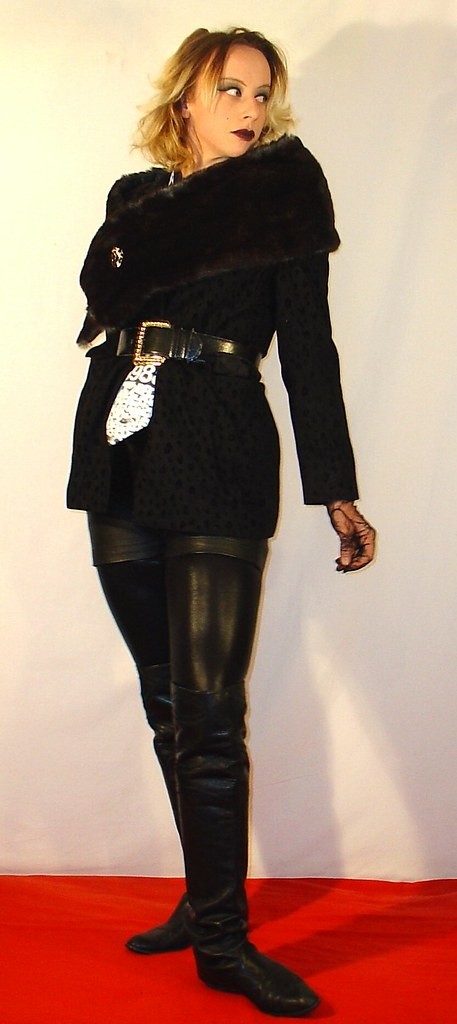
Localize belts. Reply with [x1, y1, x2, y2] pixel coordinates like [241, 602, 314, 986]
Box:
[104, 323, 263, 372]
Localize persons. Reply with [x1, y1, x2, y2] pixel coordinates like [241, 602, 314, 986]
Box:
[67, 29, 375, 1017]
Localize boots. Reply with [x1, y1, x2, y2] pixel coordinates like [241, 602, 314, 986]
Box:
[126, 666, 193, 954]
[172, 682, 320, 1017]
